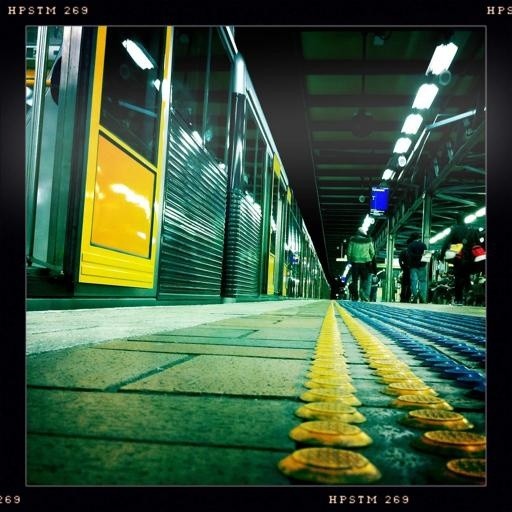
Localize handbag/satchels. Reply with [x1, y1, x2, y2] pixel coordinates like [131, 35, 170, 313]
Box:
[444, 242, 464, 262]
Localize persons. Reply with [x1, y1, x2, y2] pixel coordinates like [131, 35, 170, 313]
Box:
[441, 213, 473, 305]
[398, 239, 413, 302]
[347, 227, 375, 301]
[407, 231, 429, 303]
